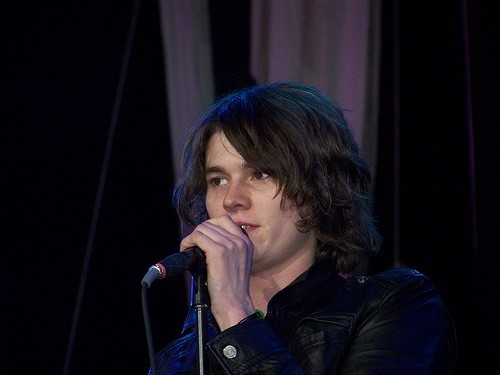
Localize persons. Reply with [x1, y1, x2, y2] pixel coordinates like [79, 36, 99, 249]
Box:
[148, 80, 443, 375]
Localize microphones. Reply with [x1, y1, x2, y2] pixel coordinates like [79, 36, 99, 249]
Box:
[142, 245, 205, 286]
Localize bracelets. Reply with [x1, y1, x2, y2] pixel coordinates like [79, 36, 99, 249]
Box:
[214, 309, 264, 342]
